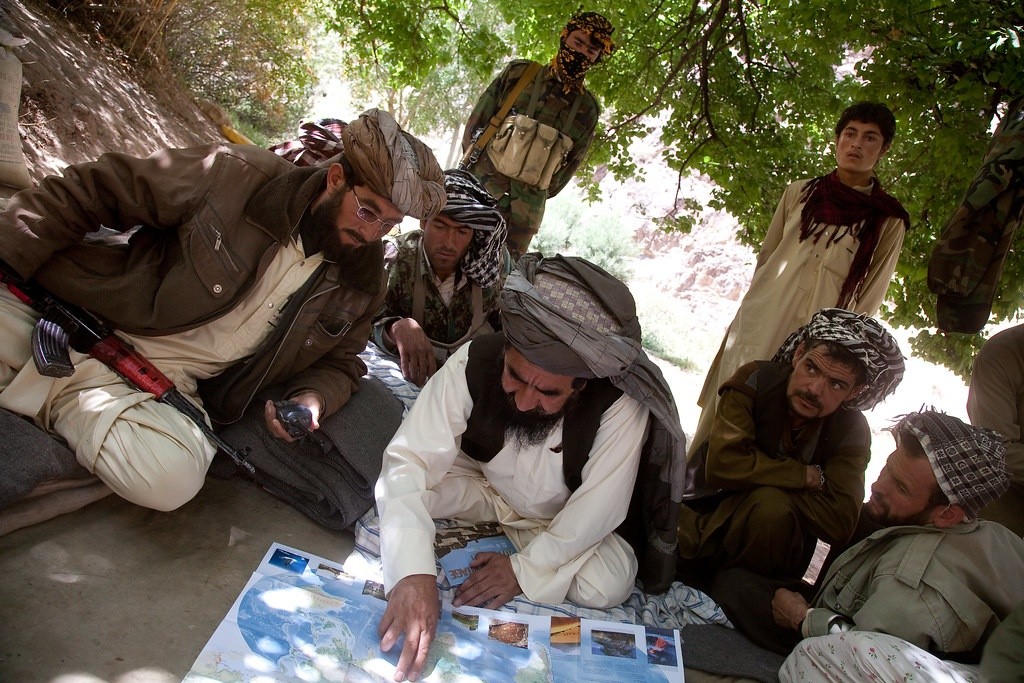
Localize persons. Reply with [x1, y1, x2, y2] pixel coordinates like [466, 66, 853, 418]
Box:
[373, 251, 687, 682]
[456, 12, 621, 263]
[965, 319, 1024, 488]
[687, 102, 908, 501]
[0, 107, 448, 514]
[773, 411, 1023, 682]
[672, 305, 905, 655]
[366, 169, 516, 389]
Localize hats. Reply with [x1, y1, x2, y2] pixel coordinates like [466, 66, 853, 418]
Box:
[341, 107, 448, 220]
[501, 251, 643, 380]
[568, 12, 615, 55]
[891, 411, 1013, 524]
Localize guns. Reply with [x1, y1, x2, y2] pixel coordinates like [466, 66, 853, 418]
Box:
[0, 259, 256, 475]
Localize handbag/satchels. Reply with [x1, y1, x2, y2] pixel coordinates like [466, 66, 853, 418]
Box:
[486, 66, 584, 191]
